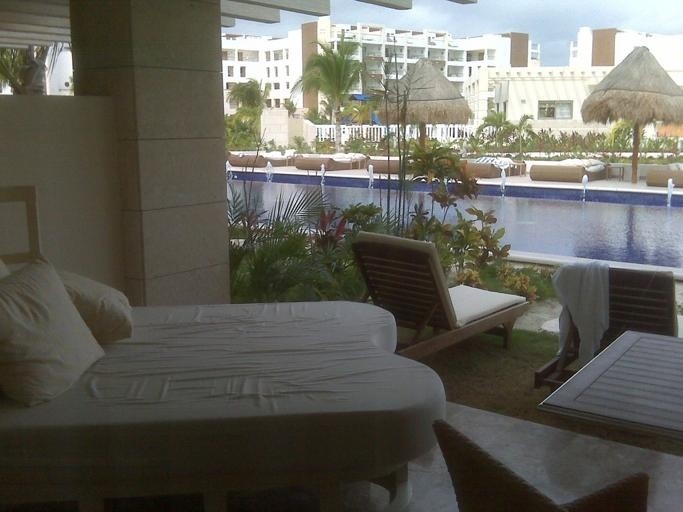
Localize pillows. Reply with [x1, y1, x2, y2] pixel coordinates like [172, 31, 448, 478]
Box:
[284, 149, 295, 157]
[0, 252, 134, 408]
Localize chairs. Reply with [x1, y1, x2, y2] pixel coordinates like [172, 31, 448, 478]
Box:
[533, 262, 679, 392]
[350, 229, 531, 360]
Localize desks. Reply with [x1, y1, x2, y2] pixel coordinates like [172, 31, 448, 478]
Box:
[535, 328, 683, 440]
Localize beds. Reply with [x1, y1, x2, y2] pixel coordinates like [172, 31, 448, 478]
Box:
[0, 299, 447, 512]
[227, 154, 683, 188]
